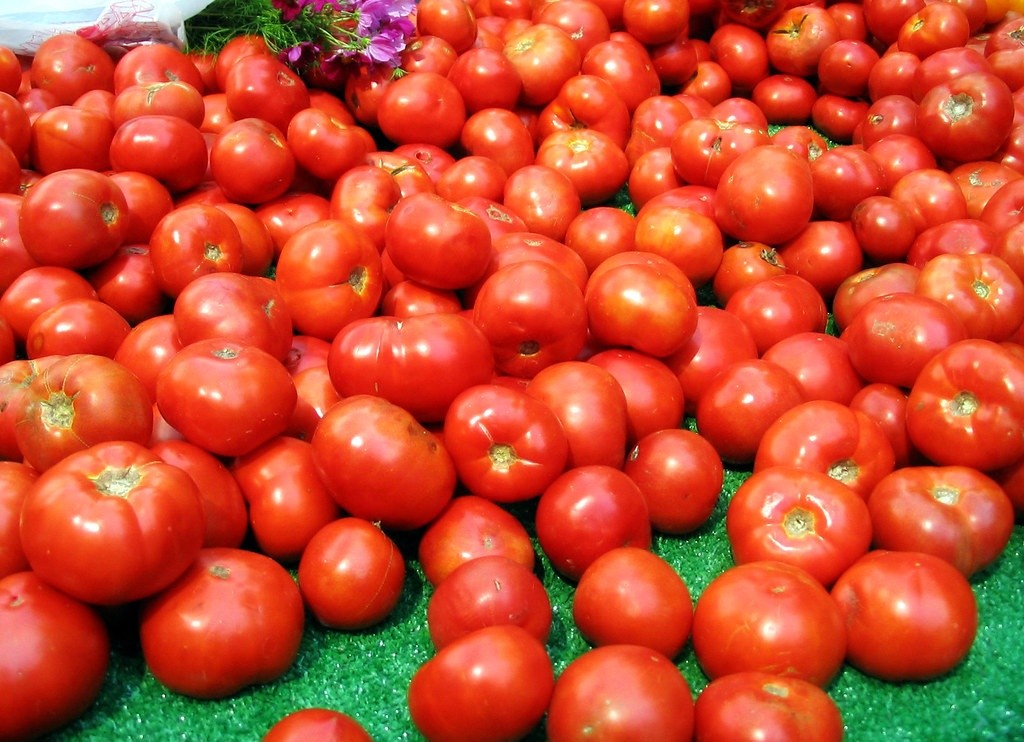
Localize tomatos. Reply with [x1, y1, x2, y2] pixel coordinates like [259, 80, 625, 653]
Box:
[0, 0, 1024, 742]
